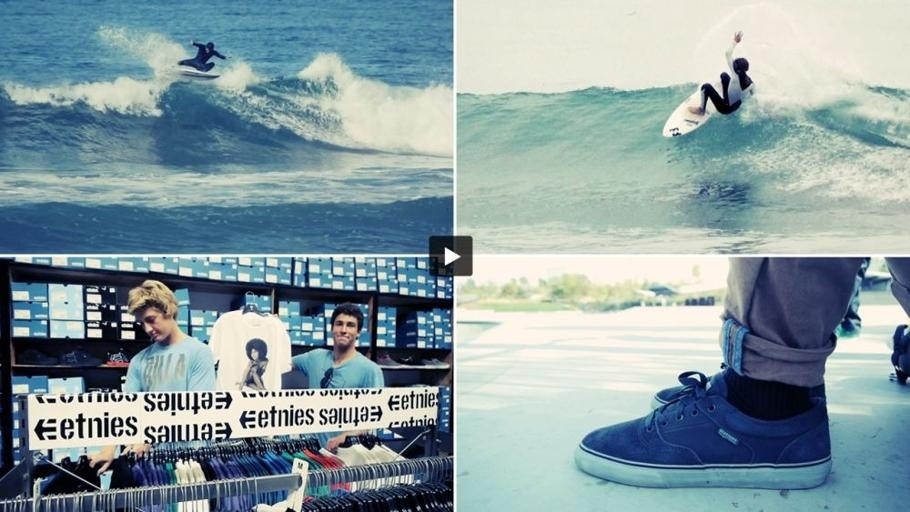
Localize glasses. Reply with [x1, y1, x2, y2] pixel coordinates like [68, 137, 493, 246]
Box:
[320, 368, 333, 388]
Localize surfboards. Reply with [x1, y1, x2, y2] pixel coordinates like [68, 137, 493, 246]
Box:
[662, 79, 725, 138]
[170, 69, 219, 81]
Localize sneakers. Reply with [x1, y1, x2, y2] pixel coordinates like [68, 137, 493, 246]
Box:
[574, 371, 833, 490]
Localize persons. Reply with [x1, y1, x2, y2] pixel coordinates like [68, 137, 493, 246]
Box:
[570, 257, 910, 488]
[287, 299, 388, 453]
[688, 30, 755, 117]
[89, 275, 217, 474]
[236, 338, 269, 390]
[175, 40, 225, 72]
